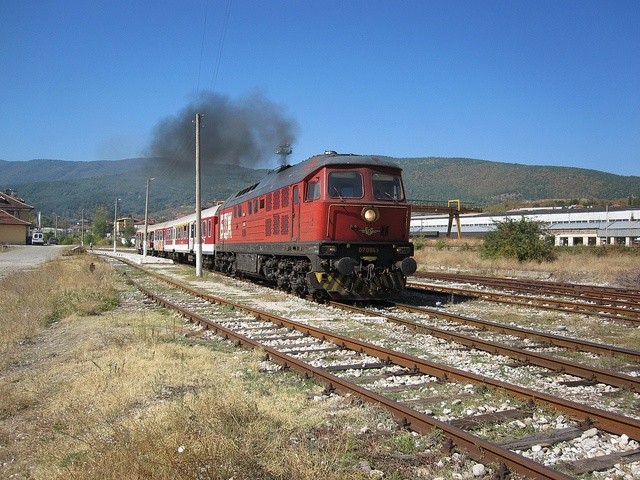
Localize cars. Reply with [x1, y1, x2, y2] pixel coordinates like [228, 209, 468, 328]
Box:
[47, 237, 58, 245]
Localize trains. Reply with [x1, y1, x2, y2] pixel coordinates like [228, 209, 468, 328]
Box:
[135, 151, 418, 305]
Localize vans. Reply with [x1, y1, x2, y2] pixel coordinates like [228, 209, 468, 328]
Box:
[32, 233, 44, 245]
